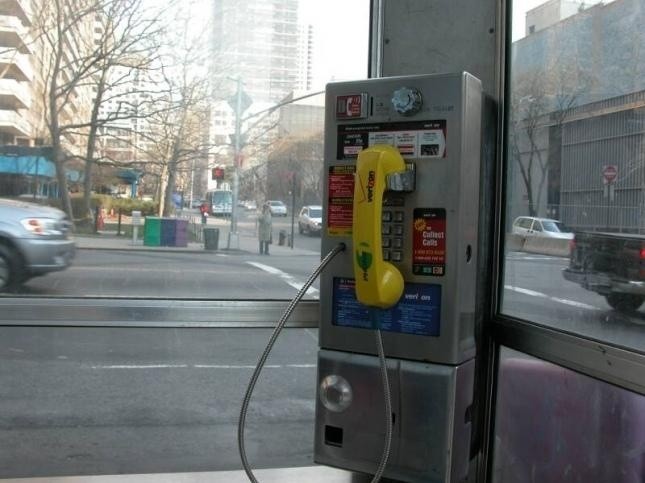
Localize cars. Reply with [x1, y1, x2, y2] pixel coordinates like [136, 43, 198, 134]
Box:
[1, 197, 77, 289]
[239, 198, 257, 210]
[299, 205, 322, 233]
[263, 201, 288, 216]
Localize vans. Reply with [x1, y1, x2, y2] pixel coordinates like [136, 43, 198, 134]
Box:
[514, 216, 576, 240]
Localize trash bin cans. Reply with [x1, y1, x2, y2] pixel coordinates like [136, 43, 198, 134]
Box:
[160, 217, 176, 247]
[203, 228, 219, 250]
[174, 219, 189, 247]
[143, 215, 161, 246]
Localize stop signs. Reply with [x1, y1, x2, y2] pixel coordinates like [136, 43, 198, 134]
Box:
[604, 165, 618, 184]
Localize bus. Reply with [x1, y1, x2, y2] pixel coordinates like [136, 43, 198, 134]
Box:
[206, 189, 233, 215]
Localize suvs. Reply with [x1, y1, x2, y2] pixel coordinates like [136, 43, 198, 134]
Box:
[561, 230, 644, 310]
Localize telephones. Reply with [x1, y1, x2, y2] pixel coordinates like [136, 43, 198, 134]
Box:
[317, 69, 498, 365]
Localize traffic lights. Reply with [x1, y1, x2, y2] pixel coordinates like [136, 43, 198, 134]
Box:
[213, 169, 223, 177]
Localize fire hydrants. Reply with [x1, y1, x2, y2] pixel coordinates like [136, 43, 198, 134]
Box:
[278, 230, 285, 245]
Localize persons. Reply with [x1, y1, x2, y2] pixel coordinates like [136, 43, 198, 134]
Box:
[200, 201, 208, 225]
[257, 199, 273, 255]
